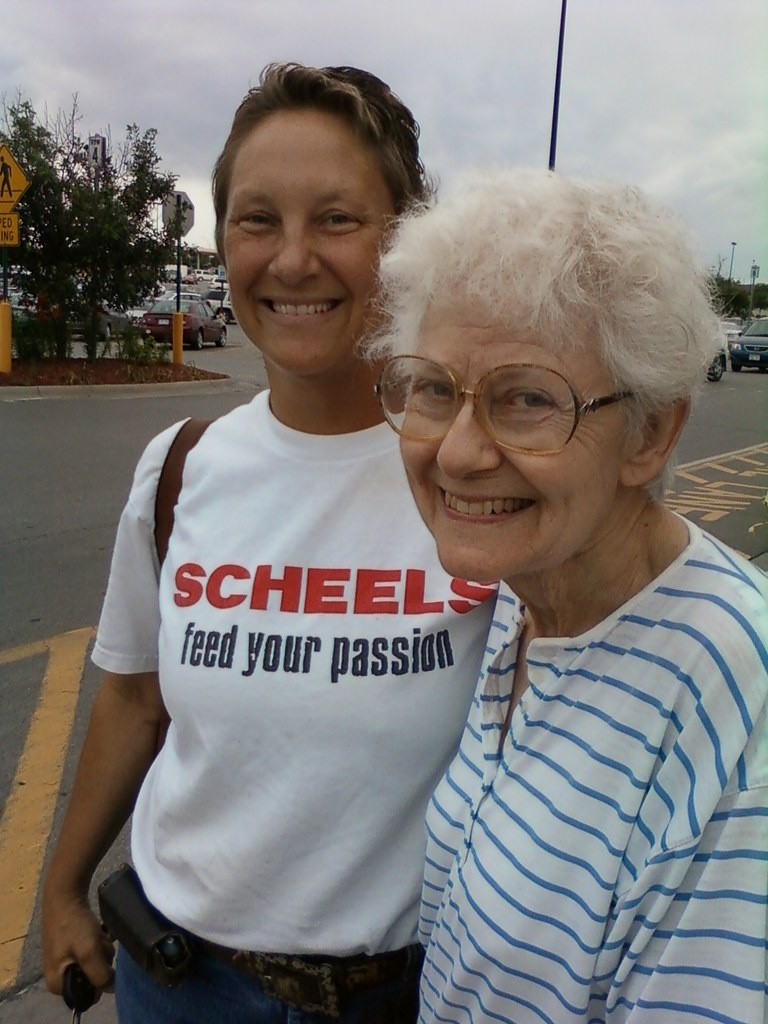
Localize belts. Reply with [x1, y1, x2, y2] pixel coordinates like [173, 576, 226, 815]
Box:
[172, 916, 431, 1019]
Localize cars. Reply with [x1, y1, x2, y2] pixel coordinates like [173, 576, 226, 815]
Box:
[125, 298, 161, 332]
[139, 299, 228, 349]
[182, 275, 197, 284]
[163, 292, 201, 315]
[707, 349, 727, 382]
[0, 265, 132, 342]
[199, 288, 236, 325]
[721, 322, 743, 350]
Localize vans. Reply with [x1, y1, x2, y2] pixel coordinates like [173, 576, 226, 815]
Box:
[191, 270, 218, 282]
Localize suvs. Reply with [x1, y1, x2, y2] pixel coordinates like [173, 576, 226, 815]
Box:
[164, 270, 182, 282]
[208, 277, 230, 290]
[729, 317, 768, 373]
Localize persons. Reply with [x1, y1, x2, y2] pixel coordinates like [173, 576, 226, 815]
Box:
[354, 161, 767, 1024]
[42, 62, 495, 1024]
[182, 268, 195, 285]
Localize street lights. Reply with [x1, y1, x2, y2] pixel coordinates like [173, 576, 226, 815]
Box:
[727, 241, 737, 282]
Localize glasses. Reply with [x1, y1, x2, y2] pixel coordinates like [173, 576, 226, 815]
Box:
[375, 348, 661, 457]
